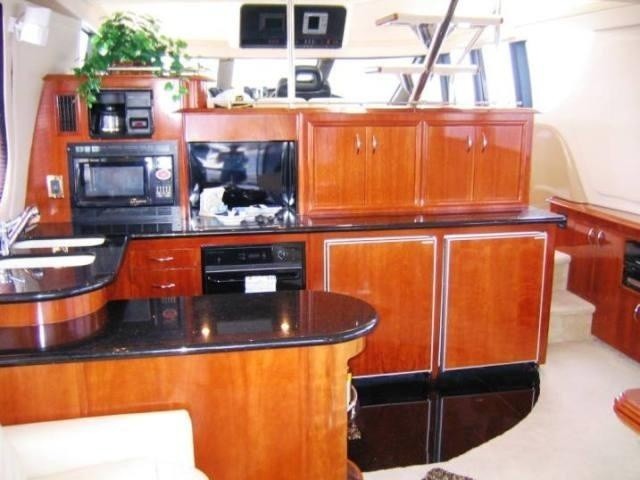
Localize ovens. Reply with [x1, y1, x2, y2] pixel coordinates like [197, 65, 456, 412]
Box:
[204, 267, 304, 291]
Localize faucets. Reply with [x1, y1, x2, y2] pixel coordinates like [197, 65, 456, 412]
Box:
[1, 205, 40, 257]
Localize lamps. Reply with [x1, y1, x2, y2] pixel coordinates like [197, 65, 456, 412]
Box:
[6, 3, 57, 48]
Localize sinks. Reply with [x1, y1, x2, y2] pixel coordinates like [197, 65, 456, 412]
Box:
[12, 233, 107, 247]
[0, 252, 97, 268]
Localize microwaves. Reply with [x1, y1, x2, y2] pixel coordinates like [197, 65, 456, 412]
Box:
[67, 143, 175, 207]
[187, 140, 296, 211]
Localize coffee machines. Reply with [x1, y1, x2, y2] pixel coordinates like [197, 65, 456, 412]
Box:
[89, 89, 153, 139]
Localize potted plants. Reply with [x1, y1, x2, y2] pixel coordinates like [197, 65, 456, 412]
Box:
[72, 11, 196, 110]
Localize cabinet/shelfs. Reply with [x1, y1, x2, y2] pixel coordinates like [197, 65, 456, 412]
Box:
[547, 198, 640, 364]
[347, 383, 534, 473]
[133, 236, 198, 299]
[303, 110, 423, 217]
[310, 219, 559, 378]
[422, 110, 534, 212]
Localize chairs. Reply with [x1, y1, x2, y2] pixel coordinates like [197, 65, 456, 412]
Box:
[0, 408, 204, 480]
